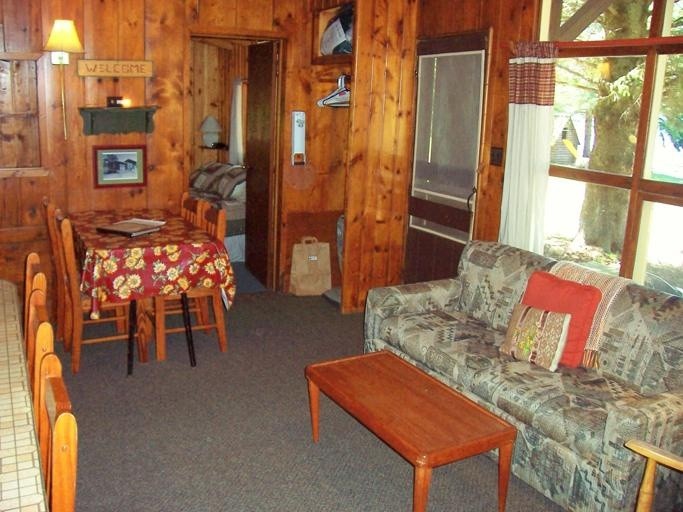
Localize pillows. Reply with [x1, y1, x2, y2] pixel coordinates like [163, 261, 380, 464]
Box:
[498, 304, 572, 371]
[520, 273, 602, 368]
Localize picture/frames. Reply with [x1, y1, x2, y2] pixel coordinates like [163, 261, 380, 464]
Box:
[92, 144, 147, 189]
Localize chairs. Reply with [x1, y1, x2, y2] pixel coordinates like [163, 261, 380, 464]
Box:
[37, 355, 78, 512]
[156, 201, 227, 360]
[26, 290, 55, 435]
[47, 202, 129, 342]
[24, 251, 47, 350]
[150, 192, 205, 325]
[53, 208, 149, 374]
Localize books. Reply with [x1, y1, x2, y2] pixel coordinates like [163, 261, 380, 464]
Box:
[96, 217, 167, 237]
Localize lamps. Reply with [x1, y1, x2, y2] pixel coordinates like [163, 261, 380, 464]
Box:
[199, 116, 222, 148]
[42, 19, 86, 65]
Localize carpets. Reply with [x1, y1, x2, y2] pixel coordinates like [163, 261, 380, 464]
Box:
[13, 292, 567, 512]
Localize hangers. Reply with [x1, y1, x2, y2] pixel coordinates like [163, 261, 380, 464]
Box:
[316, 75, 350, 107]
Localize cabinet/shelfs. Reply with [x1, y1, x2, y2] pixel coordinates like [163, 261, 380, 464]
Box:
[307, 0, 417, 314]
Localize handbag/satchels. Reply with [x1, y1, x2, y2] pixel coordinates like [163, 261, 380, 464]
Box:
[289, 242, 331, 296]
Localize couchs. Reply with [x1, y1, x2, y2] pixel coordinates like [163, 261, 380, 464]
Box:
[363, 241, 683, 512]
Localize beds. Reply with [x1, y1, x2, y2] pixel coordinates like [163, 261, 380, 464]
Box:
[173, 160, 247, 263]
